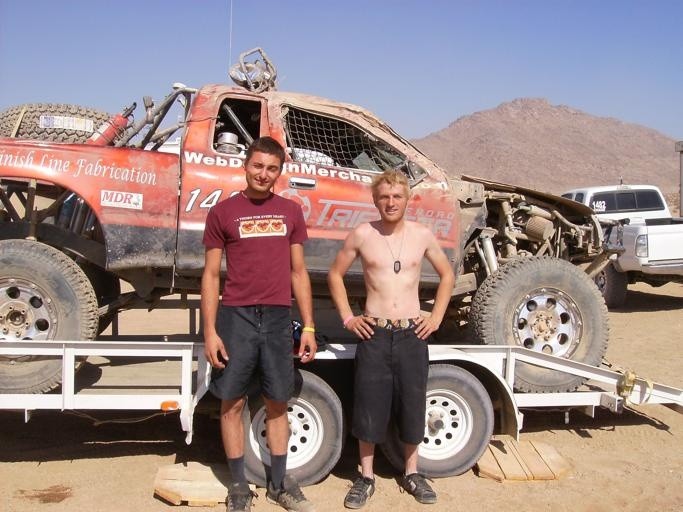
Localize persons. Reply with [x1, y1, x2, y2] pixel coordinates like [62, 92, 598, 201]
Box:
[325, 171, 455, 510]
[202, 138, 318, 511]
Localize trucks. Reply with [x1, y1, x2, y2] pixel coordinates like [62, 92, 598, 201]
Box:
[0, 325, 682, 490]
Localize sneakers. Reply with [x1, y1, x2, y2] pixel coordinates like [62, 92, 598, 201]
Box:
[267, 476, 317, 512]
[401, 472, 438, 503]
[227, 483, 253, 512]
[345, 477, 375, 509]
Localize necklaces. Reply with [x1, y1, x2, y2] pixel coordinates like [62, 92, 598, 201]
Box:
[381, 219, 404, 273]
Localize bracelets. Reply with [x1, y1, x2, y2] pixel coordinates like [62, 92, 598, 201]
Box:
[301, 327, 314, 332]
[343, 316, 353, 327]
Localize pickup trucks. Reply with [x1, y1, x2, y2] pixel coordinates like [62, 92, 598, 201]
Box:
[0, 47, 633, 397]
[548, 182, 683, 308]
